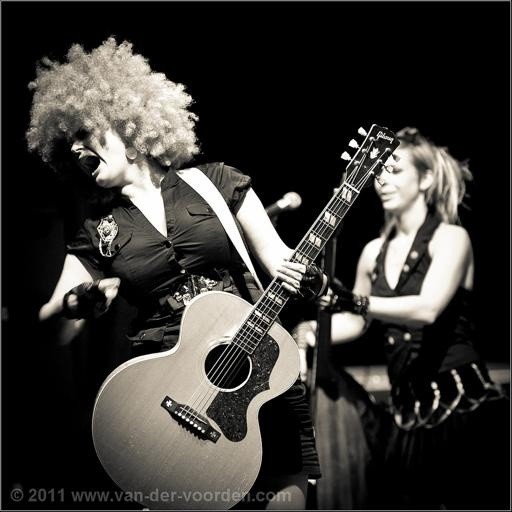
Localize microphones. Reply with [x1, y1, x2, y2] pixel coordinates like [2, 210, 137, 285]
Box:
[262, 191, 303, 217]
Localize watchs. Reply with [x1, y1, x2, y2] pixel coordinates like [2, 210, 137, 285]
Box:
[355, 295, 362, 314]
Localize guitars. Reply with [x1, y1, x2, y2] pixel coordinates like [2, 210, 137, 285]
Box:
[92, 122, 402, 512]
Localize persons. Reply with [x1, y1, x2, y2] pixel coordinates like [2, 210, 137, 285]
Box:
[291, 126, 512, 511]
[25, 37, 327, 512]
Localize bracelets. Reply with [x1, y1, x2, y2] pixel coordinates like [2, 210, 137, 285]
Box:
[361, 296, 369, 315]
